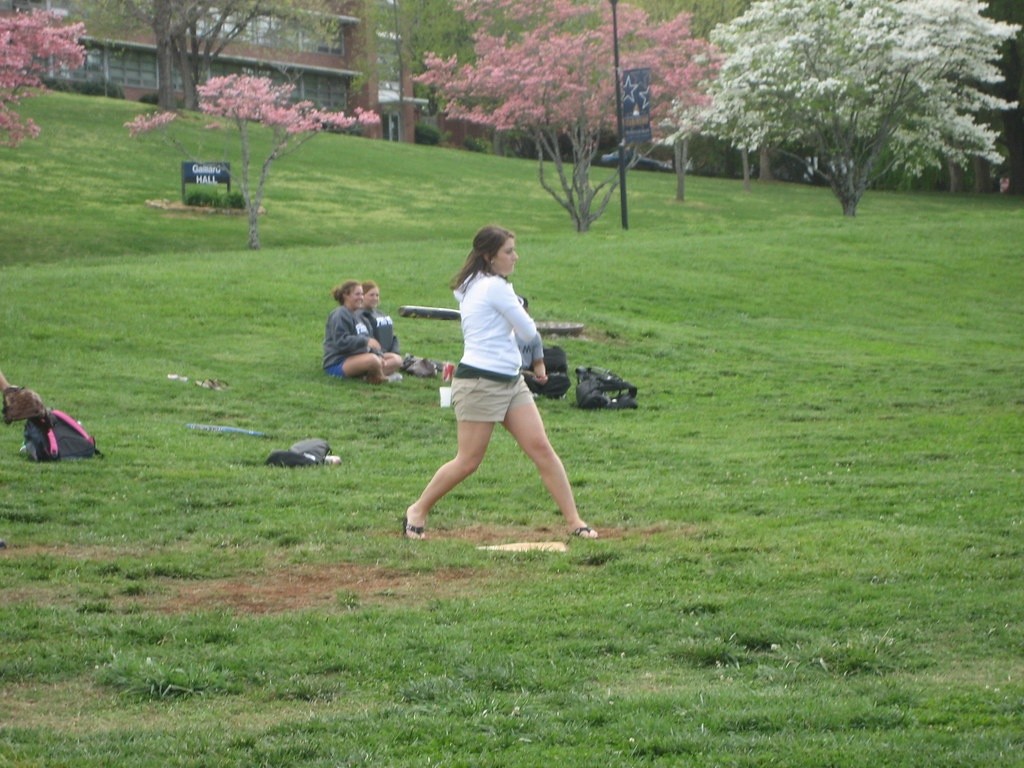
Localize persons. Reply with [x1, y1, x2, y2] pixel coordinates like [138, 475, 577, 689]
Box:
[515, 295, 547, 385]
[353, 280, 400, 355]
[402, 228, 600, 539]
[323, 279, 403, 383]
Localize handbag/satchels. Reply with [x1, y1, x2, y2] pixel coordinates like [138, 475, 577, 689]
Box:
[400, 354, 437, 377]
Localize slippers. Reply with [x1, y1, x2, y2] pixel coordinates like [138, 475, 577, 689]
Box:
[403, 517, 424, 538]
[566, 527, 598, 539]
[196, 378, 233, 391]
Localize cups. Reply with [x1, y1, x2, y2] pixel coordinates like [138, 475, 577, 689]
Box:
[439, 387, 452, 407]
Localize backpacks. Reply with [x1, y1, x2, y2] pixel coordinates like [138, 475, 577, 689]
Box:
[265, 439, 332, 467]
[19, 407, 104, 462]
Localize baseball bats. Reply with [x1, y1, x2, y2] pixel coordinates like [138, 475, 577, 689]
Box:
[396, 304, 461, 322]
[183, 422, 274, 440]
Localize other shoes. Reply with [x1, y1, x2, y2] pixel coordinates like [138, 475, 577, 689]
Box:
[386, 373, 403, 382]
